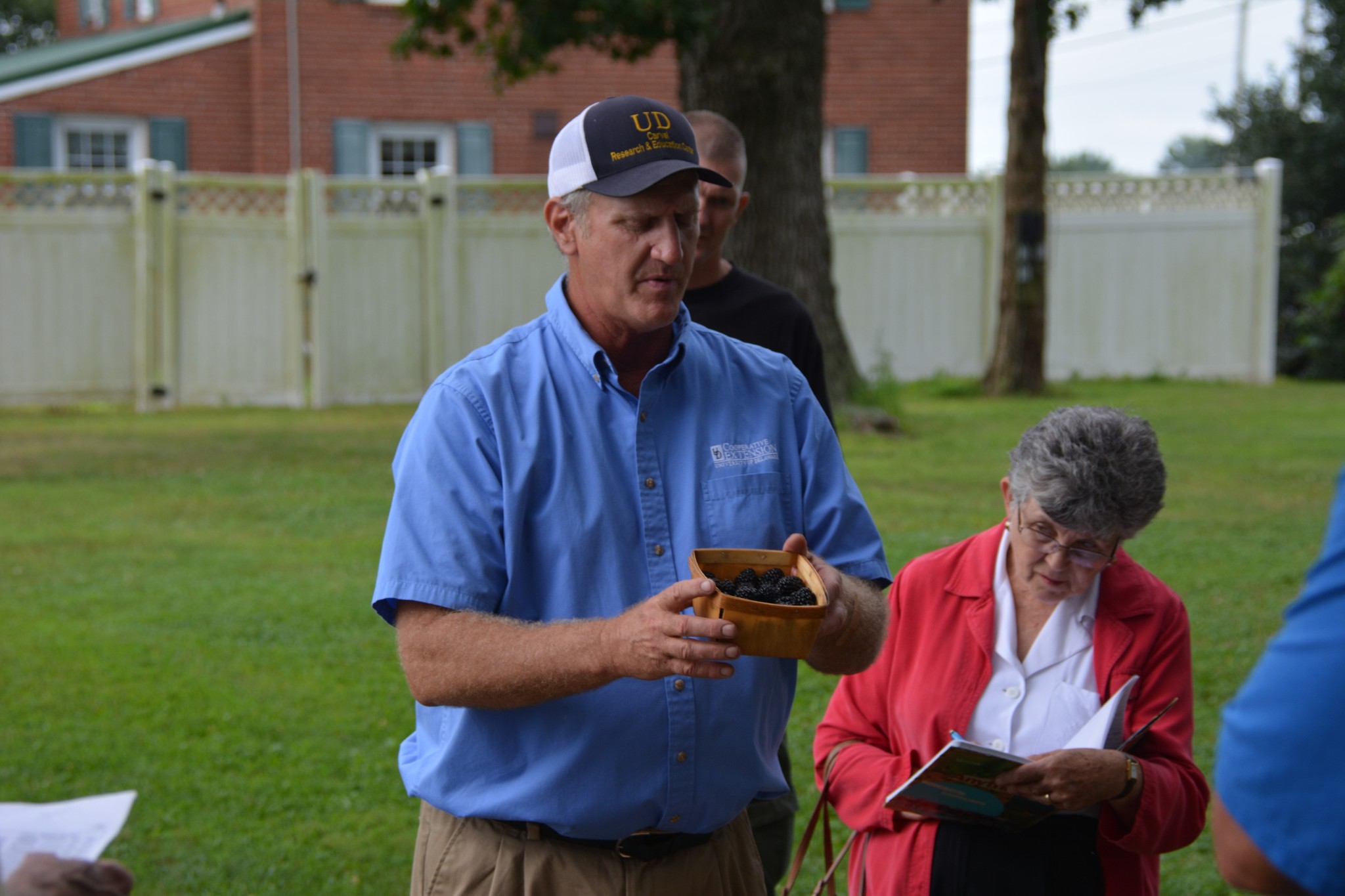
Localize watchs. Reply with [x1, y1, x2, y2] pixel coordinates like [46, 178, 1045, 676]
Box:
[1109, 749, 1142, 803]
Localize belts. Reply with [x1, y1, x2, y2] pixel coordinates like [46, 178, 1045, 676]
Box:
[506, 819, 710, 862]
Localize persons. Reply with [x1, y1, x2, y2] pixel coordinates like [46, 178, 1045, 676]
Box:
[681, 108, 838, 896]
[370, 93, 891, 896]
[1210, 471, 1345, 896]
[811, 403, 1208, 896]
[0, 852, 137, 896]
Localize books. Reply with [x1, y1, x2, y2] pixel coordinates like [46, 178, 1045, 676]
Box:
[885, 676, 1179, 826]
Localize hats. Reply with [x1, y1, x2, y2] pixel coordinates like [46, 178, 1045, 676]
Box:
[548, 94, 732, 198]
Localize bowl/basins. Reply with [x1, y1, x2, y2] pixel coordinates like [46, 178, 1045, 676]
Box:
[688, 548, 830, 659]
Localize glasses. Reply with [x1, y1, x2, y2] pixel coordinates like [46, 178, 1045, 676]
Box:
[1017, 503, 1118, 574]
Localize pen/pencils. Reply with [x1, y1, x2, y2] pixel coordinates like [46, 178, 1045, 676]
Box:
[949, 730, 963, 740]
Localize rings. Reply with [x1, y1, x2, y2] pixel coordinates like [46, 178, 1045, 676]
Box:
[1045, 793, 1050, 803]
